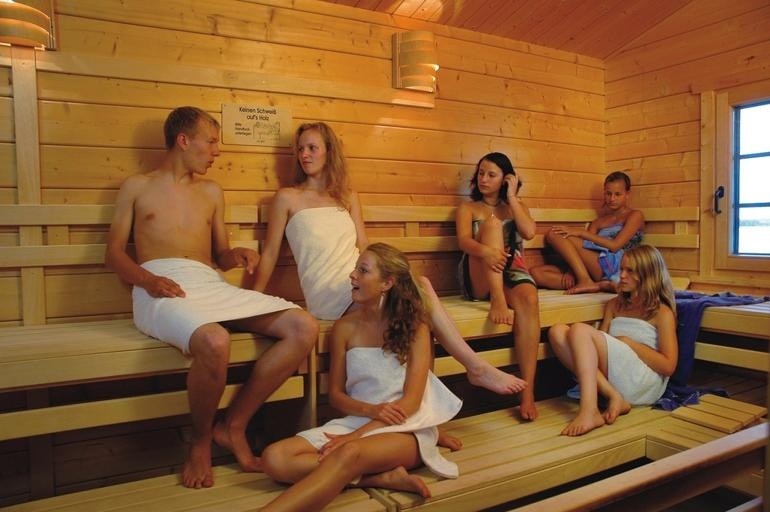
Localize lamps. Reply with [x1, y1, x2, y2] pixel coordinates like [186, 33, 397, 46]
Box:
[391, 26, 440, 94]
[1, 0, 58, 52]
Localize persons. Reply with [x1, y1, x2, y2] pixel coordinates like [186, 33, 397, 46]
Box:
[256, 241, 463, 511]
[252, 120, 532, 452]
[454, 151, 541, 421]
[546, 242, 681, 437]
[104, 106, 321, 491]
[528, 169, 647, 296]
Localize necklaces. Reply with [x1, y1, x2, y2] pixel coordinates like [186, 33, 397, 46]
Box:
[481, 197, 502, 218]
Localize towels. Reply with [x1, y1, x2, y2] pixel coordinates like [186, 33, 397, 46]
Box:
[132, 258, 305, 354]
[285, 206, 362, 320]
[297, 347, 463, 479]
[598, 316, 671, 406]
